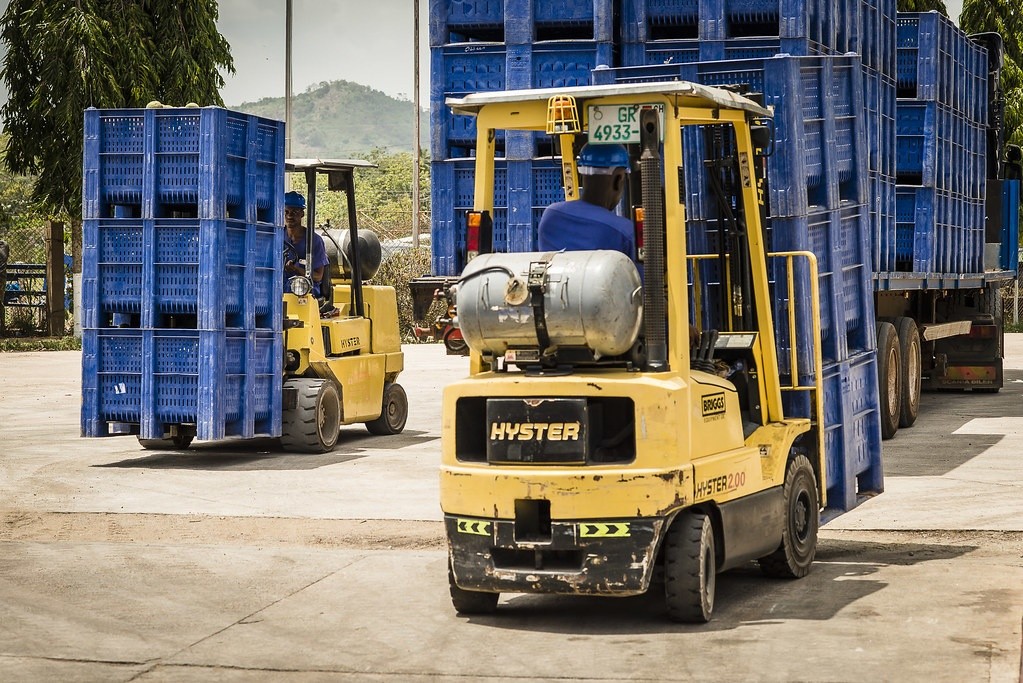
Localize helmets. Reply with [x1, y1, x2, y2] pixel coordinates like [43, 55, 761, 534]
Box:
[577, 143, 631, 175]
[285, 191, 306, 208]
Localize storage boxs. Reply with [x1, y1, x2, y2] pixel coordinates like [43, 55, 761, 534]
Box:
[78, 105, 286, 439]
[426, 0, 990, 511]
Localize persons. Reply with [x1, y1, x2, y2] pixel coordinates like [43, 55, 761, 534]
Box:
[283, 191, 330, 296]
[538, 139, 644, 287]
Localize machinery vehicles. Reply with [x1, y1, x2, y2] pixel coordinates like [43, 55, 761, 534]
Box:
[137, 158, 407, 454]
[439, 80, 826, 626]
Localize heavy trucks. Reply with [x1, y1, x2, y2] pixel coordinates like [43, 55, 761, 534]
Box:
[408, 0, 1022, 442]
